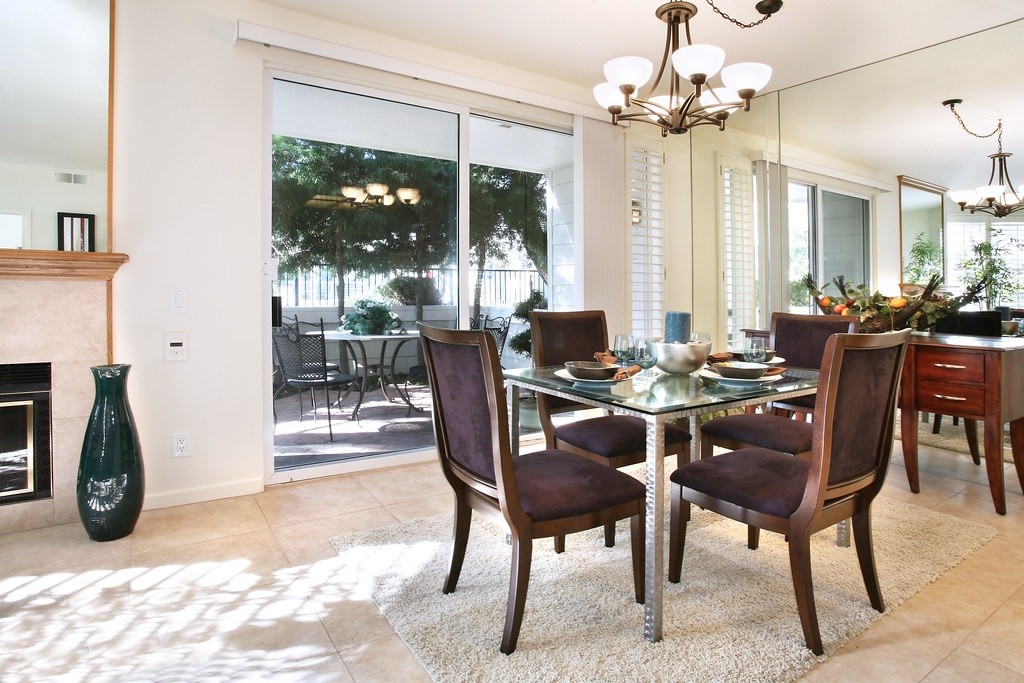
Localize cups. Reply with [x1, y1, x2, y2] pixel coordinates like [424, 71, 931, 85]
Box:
[744, 337, 766, 362]
[1012, 318, 1024, 335]
[690, 332, 711, 340]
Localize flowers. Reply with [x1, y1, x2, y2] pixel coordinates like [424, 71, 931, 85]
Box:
[336, 299, 408, 336]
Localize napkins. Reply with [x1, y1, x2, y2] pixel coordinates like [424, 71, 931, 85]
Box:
[704, 366, 788, 376]
[707, 352, 733, 366]
[613, 364, 642, 382]
[592, 350, 617, 364]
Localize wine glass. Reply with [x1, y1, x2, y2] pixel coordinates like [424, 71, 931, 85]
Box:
[614, 333, 635, 370]
[633, 338, 656, 385]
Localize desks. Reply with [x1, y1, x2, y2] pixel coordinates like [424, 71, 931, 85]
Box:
[739, 328, 1024, 516]
[304, 329, 423, 422]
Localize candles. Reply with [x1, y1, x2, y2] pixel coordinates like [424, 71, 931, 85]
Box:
[664, 311, 691, 344]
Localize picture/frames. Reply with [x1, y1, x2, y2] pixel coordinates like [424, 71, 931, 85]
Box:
[56, 212, 96, 253]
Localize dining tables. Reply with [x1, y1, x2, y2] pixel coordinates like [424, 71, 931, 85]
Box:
[501, 361, 851, 644]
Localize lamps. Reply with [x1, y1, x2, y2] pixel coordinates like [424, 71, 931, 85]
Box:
[593, 0, 783, 138]
[340, 182, 420, 210]
[941, 98, 1024, 220]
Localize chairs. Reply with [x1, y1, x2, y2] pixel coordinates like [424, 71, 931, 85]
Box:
[529, 310, 692, 548]
[667, 328, 913, 656]
[414, 320, 647, 656]
[272, 314, 363, 442]
[931, 311, 1003, 434]
[701, 312, 861, 542]
[405, 313, 510, 417]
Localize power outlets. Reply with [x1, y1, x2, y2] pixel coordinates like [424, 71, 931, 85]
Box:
[171, 432, 194, 457]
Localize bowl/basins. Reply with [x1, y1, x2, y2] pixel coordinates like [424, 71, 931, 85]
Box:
[1001, 320, 1019, 334]
[713, 362, 769, 379]
[653, 338, 712, 376]
[564, 359, 620, 380]
[726, 349, 777, 361]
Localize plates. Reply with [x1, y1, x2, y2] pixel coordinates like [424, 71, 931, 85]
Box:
[764, 357, 786, 367]
[699, 369, 781, 381]
[554, 369, 615, 383]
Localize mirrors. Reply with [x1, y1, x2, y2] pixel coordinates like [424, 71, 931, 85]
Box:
[0, 0, 116, 252]
[897, 175, 950, 285]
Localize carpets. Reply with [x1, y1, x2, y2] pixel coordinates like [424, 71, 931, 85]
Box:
[894, 408, 1015, 464]
[329, 453, 1003, 683]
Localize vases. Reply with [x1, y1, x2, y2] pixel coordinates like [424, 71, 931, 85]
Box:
[74, 363, 146, 543]
[365, 321, 385, 335]
